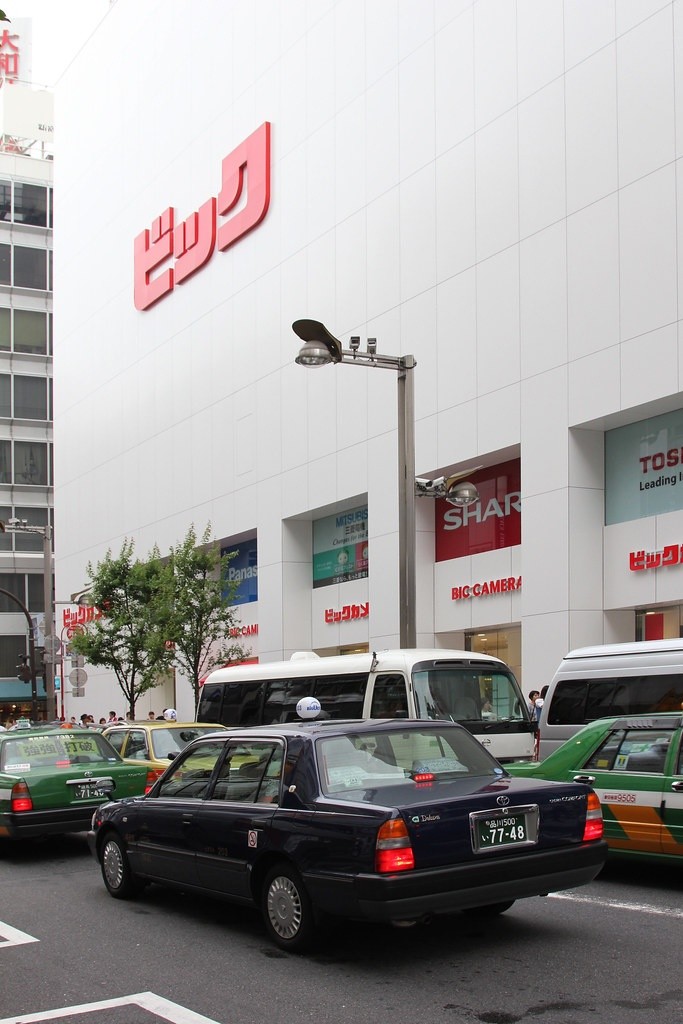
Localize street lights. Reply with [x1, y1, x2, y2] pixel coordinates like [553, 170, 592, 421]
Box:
[292, 318, 485, 650]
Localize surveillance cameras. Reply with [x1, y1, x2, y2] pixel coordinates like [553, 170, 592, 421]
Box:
[8, 517, 20, 524]
[426, 475, 448, 491]
[415, 478, 433, 487]
[367, 337, 377, 353]
[22, 519, 28, 525]
[349, 336, 360, 350]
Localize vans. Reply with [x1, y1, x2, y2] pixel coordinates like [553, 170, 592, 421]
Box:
[537, 637, 683, 763]
[193, 648, 538, 767]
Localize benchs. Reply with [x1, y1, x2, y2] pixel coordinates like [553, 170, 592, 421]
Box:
[329, 757, 469, 790]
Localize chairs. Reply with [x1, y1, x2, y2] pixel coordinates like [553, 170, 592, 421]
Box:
[454, 696, 480, 719]
[225, 762, 270, 799]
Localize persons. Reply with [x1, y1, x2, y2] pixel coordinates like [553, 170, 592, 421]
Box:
[156, 708, 167, 720]
[147, 711, 156, 720]
[535, 685, 550, 721]
[527, 690, 540, 721]
[54, 711, 131, 728]
[3, 716, 43, 728]
[480, 697, 497, 719]
[264, 749, 316, 869]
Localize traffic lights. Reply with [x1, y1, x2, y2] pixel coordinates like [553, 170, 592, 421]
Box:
[16, 664, 31, 683]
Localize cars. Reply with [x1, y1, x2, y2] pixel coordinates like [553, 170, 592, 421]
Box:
[500, 711, 683, 864]
[86, 716, 608, 952]
[0, 718, 261, 841]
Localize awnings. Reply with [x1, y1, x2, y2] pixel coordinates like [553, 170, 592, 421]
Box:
[0, 679, 46, 703]
